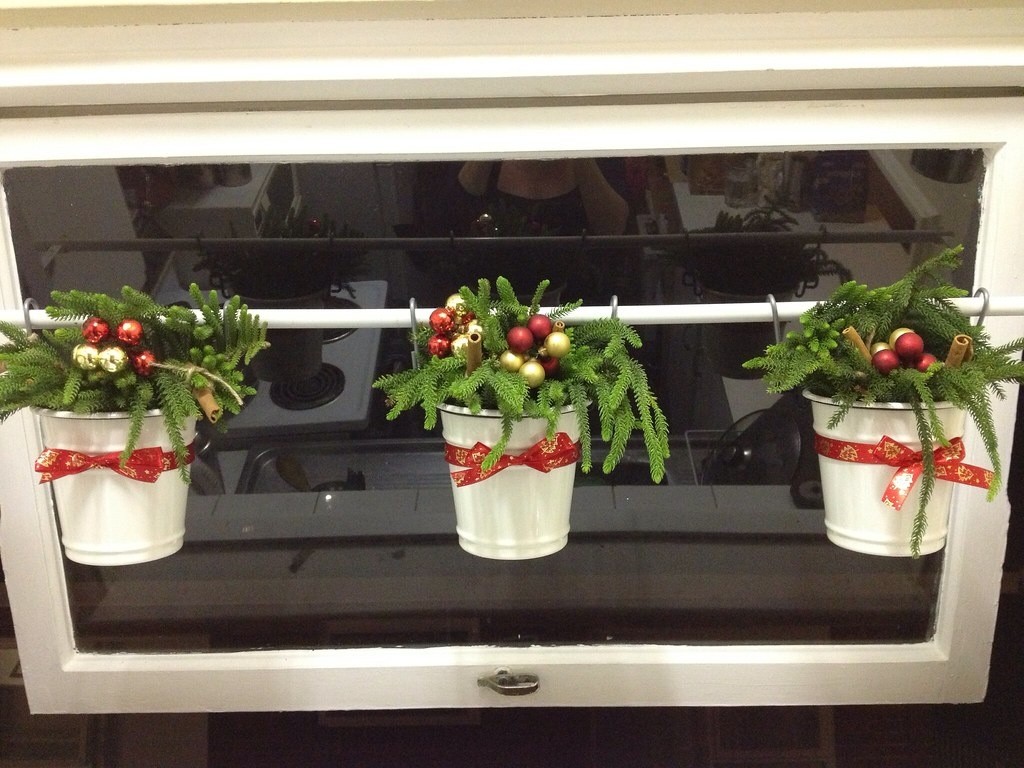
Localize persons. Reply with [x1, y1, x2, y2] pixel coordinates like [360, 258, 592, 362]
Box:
[445, 160, 638, 314]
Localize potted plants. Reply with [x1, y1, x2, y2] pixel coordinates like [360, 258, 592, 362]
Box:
[660, 191, 852, 384]
[740, 242, 1024, 566]
[187, 202, 362, 384]
[0, 283, 276, 569]
[418, 208, 584, 302]
[351, 276, 675, 561]
